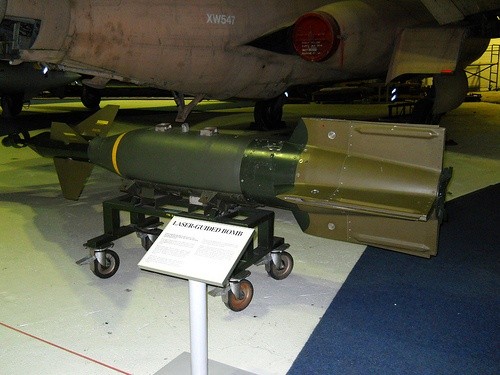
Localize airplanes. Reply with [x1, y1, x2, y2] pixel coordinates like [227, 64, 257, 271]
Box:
[1, 0, 499, 145]
